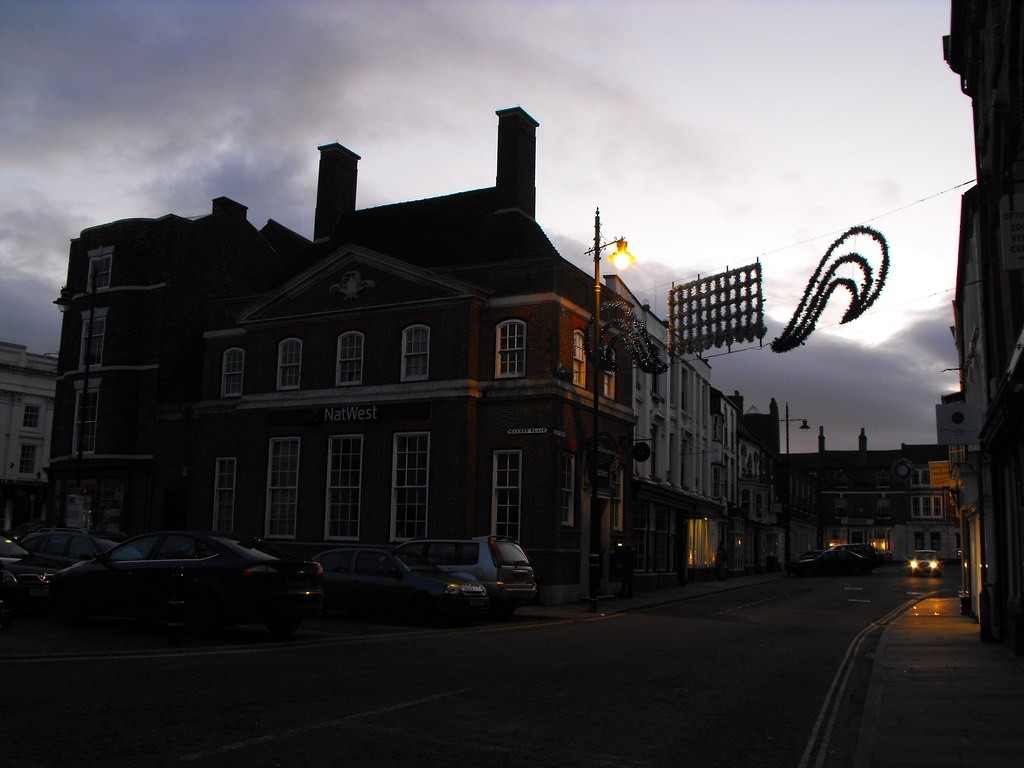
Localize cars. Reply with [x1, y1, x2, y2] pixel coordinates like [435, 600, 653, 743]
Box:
[907, 550, 945, 577]
[311, 546, 489, 628]
[0, 529, 326, 641]
[790, 544, 892, 577]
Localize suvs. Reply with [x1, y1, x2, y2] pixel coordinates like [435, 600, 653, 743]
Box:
[376, 534, 541, 621]
[10, 527, 144, 570]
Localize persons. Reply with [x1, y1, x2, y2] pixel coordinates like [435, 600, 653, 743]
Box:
[614, 538, 635, 599]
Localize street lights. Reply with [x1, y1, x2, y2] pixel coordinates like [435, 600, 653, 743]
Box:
[778, 402, 811, 578]
[570, 208, 636, 614]
[53, 264, 98, 459]
